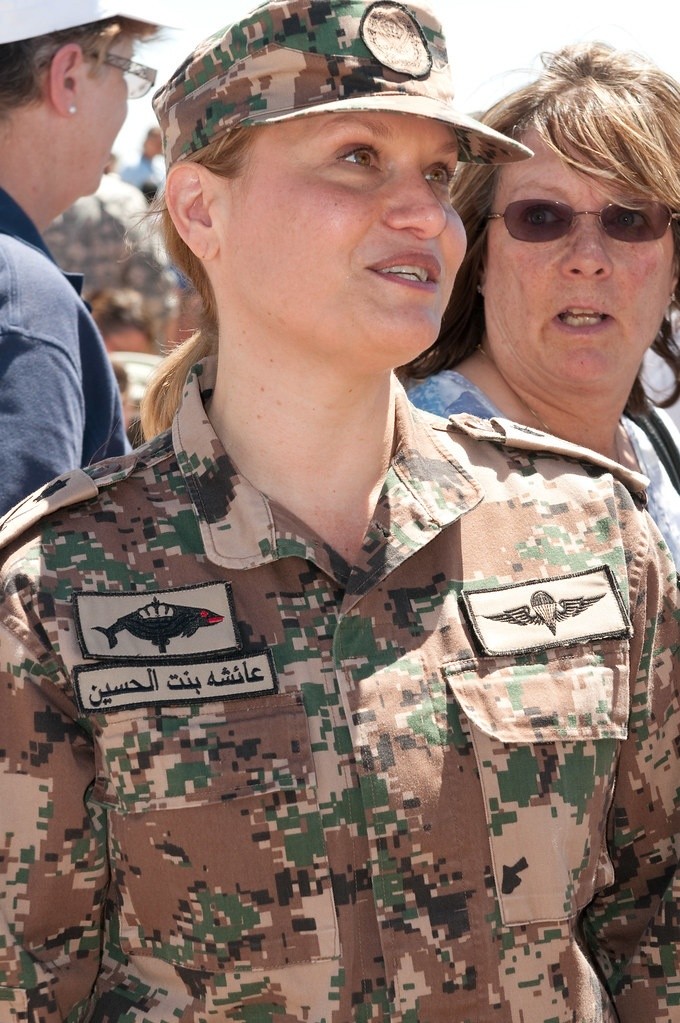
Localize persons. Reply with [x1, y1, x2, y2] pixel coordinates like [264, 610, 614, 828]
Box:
[0, 0, 680, 1023]
[404, 40, 680, 581]
[0, 0, 193, 522]
[84, 286, 158, 447]
[42, 153, 180, 345]
[121, 127, 166, 192]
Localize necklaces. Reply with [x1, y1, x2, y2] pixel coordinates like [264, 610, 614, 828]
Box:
[479, 345, 557, 437]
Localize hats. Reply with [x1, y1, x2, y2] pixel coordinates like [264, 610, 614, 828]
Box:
[151, 0, 534, 180]
[0, 0, 179, 47]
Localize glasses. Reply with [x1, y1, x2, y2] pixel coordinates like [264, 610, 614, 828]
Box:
[38, 48, 157, 99]
[485, 198, 680, 243]
